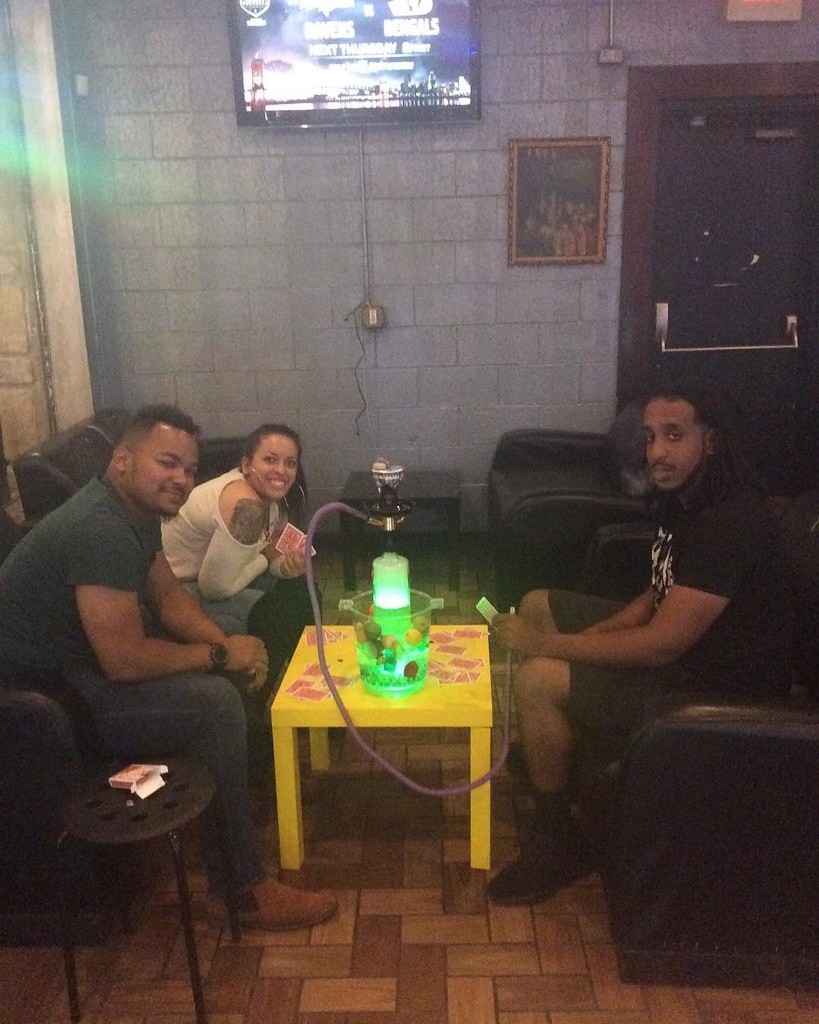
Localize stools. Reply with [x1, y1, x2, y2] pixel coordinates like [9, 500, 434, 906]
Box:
[49, 762, 243, 1024]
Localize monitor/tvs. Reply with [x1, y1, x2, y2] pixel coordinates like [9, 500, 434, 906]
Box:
[226, 0, 482, 128]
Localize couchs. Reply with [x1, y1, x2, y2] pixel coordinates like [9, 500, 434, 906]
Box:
[12, 410, 308, 533]
[486, 397, 683, 616]
[573, 491, 819, 983]
[0, 511, 171, 945]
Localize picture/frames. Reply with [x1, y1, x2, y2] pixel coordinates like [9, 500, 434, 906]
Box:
[507, 136, 611, 265]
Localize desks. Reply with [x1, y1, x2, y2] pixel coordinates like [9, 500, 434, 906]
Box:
[268, 623, 493, 869]
[339, 469, 460, 593]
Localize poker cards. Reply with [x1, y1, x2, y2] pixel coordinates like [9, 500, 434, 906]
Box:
[475, 595, 502, 630]
[288, 620, 490, 704]
[275, 523, 317, 560]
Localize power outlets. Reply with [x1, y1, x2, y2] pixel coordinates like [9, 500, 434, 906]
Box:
[361, 306, 385, 329]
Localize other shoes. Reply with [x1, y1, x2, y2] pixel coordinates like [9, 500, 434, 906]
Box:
[213, 874, 338, 930]
[488, 834, 580, 905]
[507, 741, 533, 775]
[247, 768, 327, 806]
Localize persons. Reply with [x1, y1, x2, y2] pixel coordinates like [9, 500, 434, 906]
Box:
[160, 423, 347, 741]
[489, 370, 794, 905]
[1, 405, 339, 931]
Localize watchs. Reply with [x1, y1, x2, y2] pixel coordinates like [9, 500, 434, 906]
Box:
[208, 640, 228, 674]
